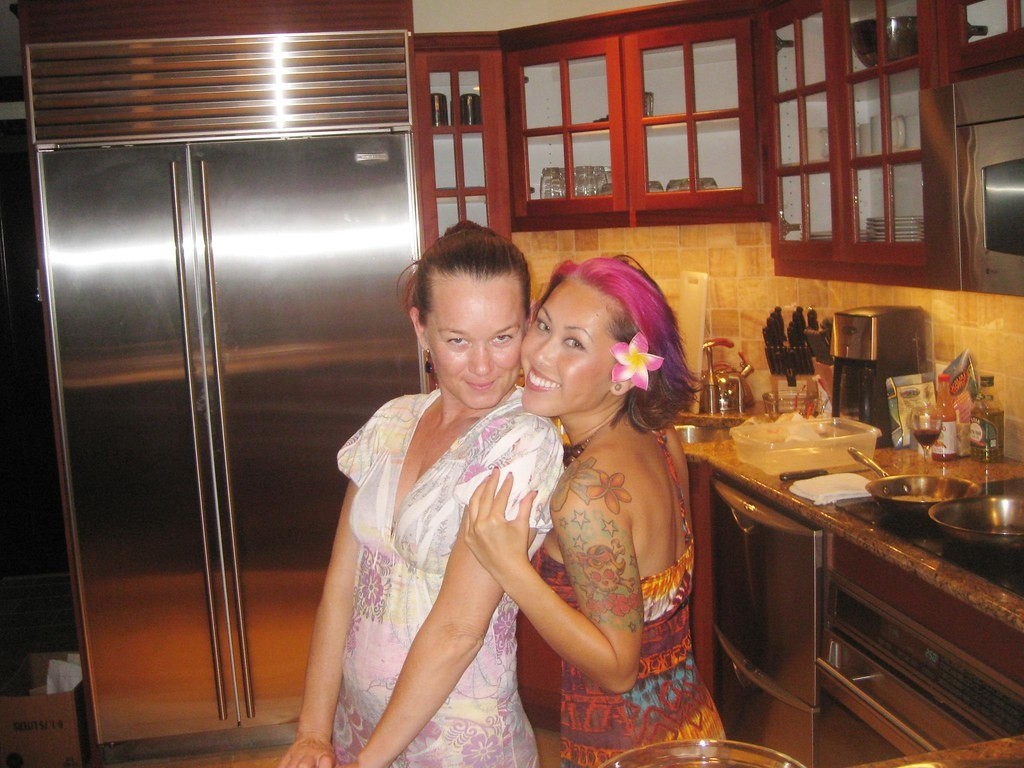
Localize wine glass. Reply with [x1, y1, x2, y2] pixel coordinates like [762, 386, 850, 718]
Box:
[910, 406, 941, 475]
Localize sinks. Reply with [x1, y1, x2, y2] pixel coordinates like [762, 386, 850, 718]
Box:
[672, 423, 736, 445]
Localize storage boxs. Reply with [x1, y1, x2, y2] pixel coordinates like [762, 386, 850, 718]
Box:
[0, 651, 92, 768]
[728, 417, 883, 477]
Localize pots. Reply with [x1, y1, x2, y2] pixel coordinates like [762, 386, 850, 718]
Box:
[848, 447, 983, 522]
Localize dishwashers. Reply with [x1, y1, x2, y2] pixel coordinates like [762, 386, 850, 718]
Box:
[709, 474, 824, 768]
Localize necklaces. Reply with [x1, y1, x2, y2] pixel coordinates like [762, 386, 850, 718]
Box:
[563, 437, 590, 471]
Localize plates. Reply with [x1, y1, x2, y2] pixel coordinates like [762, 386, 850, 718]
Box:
[800, 217, 924, 242]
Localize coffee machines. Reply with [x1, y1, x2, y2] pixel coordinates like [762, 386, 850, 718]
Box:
[829, 305, 928, 449]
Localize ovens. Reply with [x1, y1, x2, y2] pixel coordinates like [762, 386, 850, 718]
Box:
[919, 69, 1024, 296]
[814, 631, 986, 768]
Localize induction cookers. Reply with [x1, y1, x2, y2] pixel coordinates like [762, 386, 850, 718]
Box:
[835, 477, 1023, 599]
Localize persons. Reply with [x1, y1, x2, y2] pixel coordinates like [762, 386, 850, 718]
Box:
[280, 221, 560, 768]
[466, 255, 728, 768]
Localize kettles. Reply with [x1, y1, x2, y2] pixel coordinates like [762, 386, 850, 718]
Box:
[700, 336, 754, 415]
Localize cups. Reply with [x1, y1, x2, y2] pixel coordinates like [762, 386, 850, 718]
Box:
[540, 166, 717, 199]
[806, 114, 919, 169]
[644, 92, 654, 116]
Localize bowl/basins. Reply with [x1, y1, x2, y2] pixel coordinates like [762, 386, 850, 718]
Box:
[598, 738, 807, 768]
[848, 16, 918, 68]
[761, 390, 818, 423]
[928, 494, 1023, 552]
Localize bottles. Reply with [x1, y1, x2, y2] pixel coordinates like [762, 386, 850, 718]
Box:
[970, 375, 1004, 464]
[931, 374, 957, 462]
[776, 35, 794, 52]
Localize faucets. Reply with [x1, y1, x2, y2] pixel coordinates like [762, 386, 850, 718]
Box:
[704, 345, 720, 414]
[727, 363, 755, 415]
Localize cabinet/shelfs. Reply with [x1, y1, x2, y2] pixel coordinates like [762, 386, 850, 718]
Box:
[516, 462, 1024, 768]
[415, 0, 1024, 297]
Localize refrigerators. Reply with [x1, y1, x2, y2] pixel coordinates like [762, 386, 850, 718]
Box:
[30, 127, 427, 751]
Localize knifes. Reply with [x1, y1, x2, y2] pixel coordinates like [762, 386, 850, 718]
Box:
[779, 463, 888, 481]
[762, 306, 835, 390]
